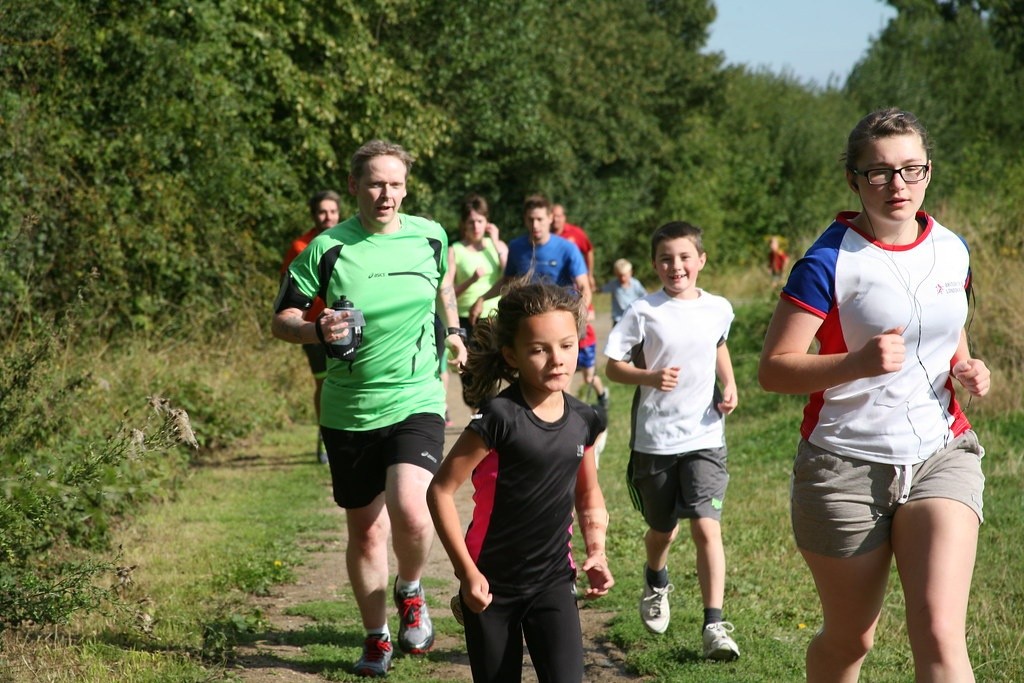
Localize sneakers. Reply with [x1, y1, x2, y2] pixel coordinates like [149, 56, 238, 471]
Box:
[394, 575, 436, 657]
[701, 620, 741, 662]
[353, 633, 393, 676]
[641, 560, 676, 635]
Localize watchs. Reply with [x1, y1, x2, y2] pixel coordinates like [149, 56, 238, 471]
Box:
[444, 327, 467, 342]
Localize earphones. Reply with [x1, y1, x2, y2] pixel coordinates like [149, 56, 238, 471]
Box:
[851, 178, 859, 192]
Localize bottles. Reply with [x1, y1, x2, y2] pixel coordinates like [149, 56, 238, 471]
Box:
[327, 295, 362, 361]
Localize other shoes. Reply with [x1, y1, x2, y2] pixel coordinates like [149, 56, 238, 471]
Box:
[598, 386, 610, 411]
[318, 432, 330, 465]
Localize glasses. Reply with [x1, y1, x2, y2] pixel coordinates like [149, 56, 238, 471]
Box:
[851, 161, 931, 186]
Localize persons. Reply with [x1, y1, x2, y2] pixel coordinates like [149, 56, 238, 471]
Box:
[470, 195, 592, 325]
[286, 191, 340, 462]
[768, 241, 790, 290]
[417, 212, 451, 426]
[594, 257, 648, 327]
[604, 220, 741, 661]
[427, 282, 614, 683]
[272, 140, 468, 678]
[576, 305, 610, 409]
[447, 198, 508, 418]
[760, 107, 991, 683]
[551, 204, 596, 290]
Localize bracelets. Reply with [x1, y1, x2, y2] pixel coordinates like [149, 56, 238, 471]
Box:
[498, 251, 505, 257]
